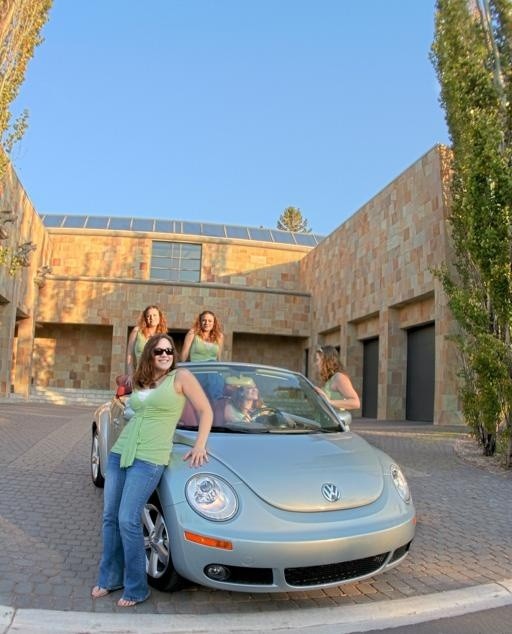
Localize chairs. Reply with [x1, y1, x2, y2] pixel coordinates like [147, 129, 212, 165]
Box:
[213, 384, 239, 427]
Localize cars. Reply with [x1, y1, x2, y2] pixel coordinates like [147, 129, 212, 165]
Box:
[89, 361, 418, 594]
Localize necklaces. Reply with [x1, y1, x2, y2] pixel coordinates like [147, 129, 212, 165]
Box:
[148, 372, 167, 388]
[244, 407, 252, 412]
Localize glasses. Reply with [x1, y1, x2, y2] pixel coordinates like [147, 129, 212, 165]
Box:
[154, 347, 174, 355]
[317, 346, 323, 352]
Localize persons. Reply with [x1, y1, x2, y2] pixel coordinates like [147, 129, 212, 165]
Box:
[90, 333, 215, 607]
[224, 377, 274, 424]
[180, 310, 226, 402]
[125, 305, 169, 388]
[223, 376, 240, 396]
[314, 344, 361, 431]
